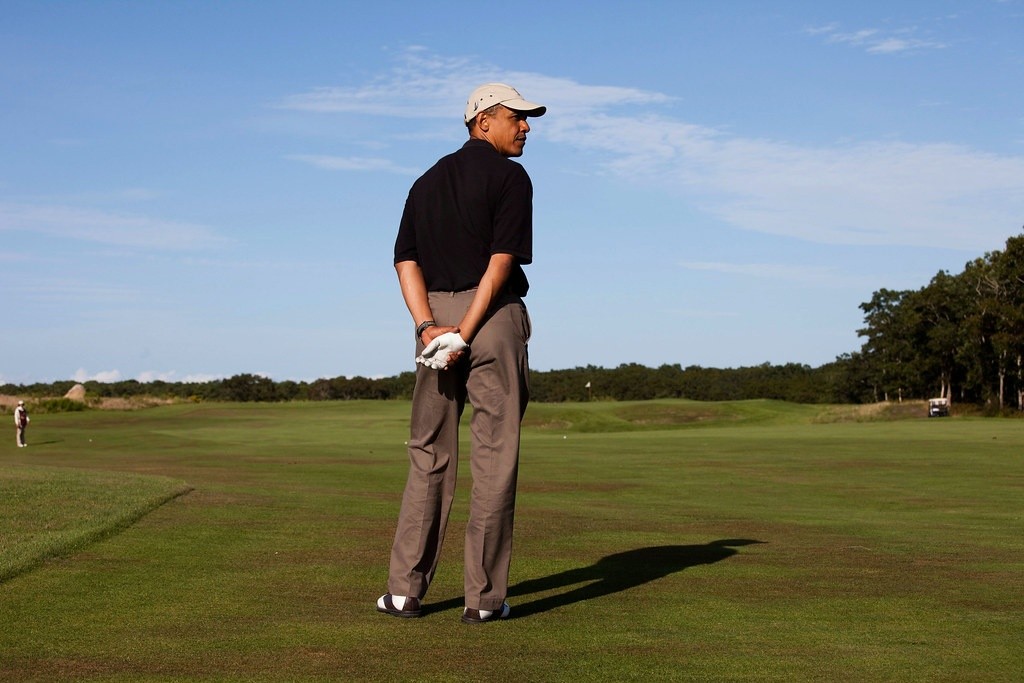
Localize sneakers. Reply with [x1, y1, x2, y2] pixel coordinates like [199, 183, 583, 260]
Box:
[376, 592, 421, 618]
[462, 603, 510, 624]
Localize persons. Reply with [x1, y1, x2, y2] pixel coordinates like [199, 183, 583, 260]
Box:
[14, 400, 30, 447]
[375, 82, 548, 620]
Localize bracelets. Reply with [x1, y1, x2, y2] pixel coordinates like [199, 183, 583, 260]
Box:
[417, 321, 436, 340]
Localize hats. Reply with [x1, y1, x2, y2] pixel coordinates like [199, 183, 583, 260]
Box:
[464, 83, 547, 127]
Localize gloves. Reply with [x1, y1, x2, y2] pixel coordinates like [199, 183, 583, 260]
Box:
[416, 331, 469, 370]
